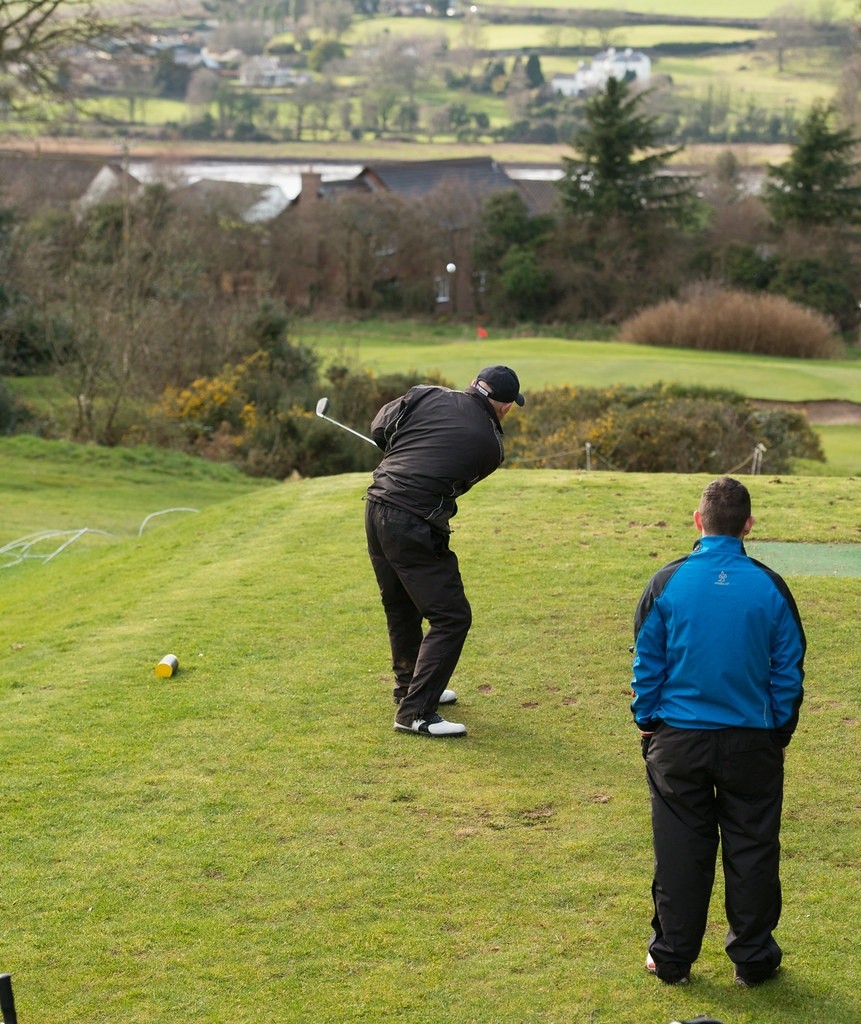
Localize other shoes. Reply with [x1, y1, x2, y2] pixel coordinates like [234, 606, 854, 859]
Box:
[646, 953, 687, 984]
[732, 964, 781, 989]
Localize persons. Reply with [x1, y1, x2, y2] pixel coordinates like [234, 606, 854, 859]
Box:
[630, 477, 807, 990]
[365, 366, 525, 735]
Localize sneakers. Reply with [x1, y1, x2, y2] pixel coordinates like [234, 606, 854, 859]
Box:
[394, 712, 468, 737]
[394, 689, 458, 705]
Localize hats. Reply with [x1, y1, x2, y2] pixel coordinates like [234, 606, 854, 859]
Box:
[475, 365, 525, 406]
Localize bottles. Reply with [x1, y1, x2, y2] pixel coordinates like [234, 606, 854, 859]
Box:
[156, 654, 178, 677]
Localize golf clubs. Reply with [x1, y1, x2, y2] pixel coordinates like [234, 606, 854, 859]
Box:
[314, 396, 378, 447]
